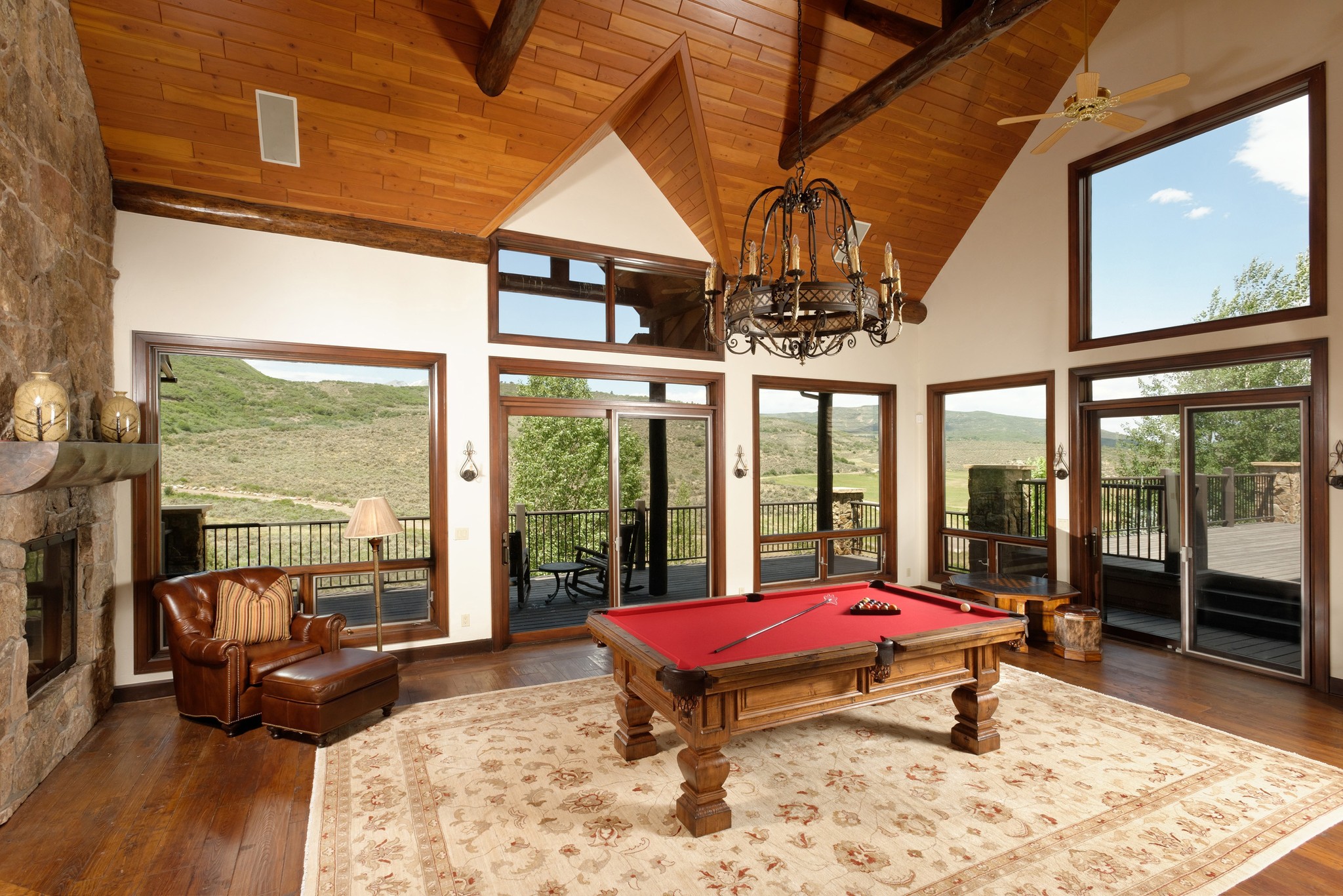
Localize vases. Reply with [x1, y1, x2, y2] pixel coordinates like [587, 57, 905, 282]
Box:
[14, 372, 70, 442]
[102, 391, 140, 443]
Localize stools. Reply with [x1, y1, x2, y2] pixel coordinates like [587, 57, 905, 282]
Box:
[265, 647, 399, 743]
[1054, 604, 1102, 662]
[942, 580, 956, 596]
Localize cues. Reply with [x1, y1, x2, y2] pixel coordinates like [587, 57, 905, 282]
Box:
[714, 598, 832, 653]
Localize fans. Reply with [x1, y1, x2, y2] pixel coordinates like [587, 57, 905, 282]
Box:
[996, 0, 1191, 155]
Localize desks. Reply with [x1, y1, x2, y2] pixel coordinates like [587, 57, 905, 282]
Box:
[539, 560, 585, 603]
[585, 581, 1026, 836]
[951, 572, 1080, 652]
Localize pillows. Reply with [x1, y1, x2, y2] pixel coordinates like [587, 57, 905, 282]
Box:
[214, 571, 293, 645]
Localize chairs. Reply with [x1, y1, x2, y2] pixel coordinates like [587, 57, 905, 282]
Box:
[568, 520, 644, 597]
[509, 530, 531, 607]
[153, 565, 346, 738]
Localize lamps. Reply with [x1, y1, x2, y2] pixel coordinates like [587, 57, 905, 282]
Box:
[342, 497, 405, 651]
[734, 444, 749, 478]
[699, 0, 908, 366]
[1327, 440, 1343, 490]
[1053, 443, 1070, 479]
[460, 439, 479, 481]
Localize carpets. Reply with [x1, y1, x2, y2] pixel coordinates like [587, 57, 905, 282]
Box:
[298, 660, 1343, 896]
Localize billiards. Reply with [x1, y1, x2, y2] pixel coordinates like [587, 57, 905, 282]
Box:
[960, 603, 971, 612]
[852, 597, 897, 611]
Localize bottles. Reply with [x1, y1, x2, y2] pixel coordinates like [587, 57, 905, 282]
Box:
[13, 371, 71, 443]
[100, 391, 141, 445]
